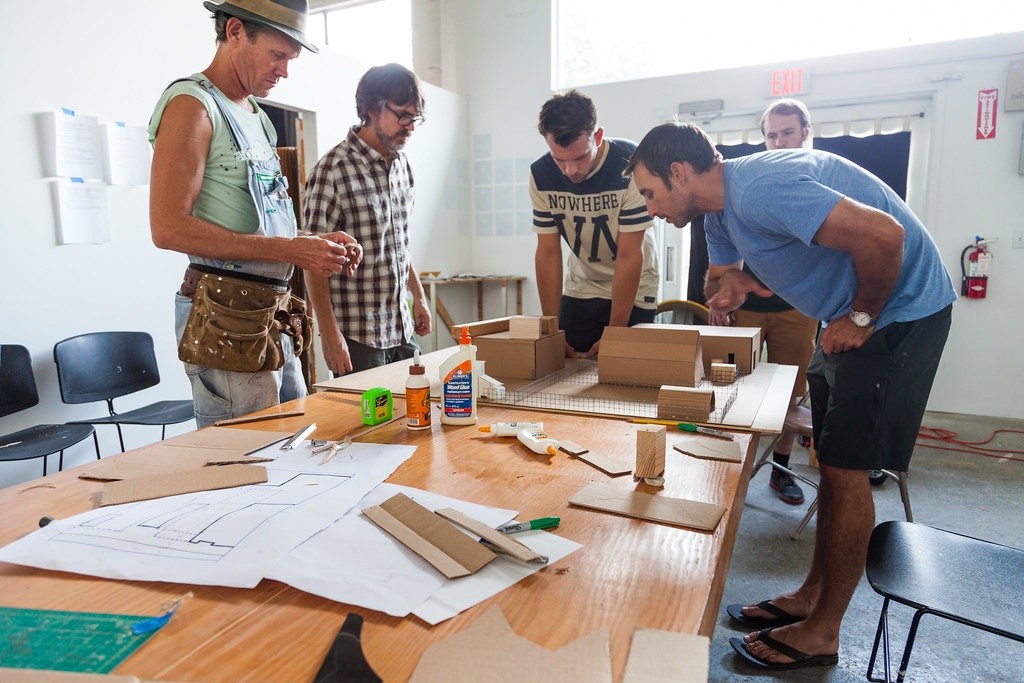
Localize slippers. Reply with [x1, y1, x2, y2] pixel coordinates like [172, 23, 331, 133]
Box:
[726, 598, 808, 624]
[728, 624, 839, 669]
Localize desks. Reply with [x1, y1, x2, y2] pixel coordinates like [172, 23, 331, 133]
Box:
[420, 276, 530, 354]
[0, 354, 759, 683]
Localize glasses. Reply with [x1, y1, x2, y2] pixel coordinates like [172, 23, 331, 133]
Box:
[381, 101, 426, 128]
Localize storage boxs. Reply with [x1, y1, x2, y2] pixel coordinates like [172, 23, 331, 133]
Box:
[597, 322, 762, 390]
[464, 314, 567, 381]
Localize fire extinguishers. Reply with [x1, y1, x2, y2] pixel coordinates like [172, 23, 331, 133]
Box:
[960, 236, 996, 298]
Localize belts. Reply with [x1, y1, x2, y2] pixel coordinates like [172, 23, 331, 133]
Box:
[181, 267, 288, 301]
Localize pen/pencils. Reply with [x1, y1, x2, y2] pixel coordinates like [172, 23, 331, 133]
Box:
[627, 417, 681, 426]
[479, 517, 560, 543]
[678, 423, 734, 438]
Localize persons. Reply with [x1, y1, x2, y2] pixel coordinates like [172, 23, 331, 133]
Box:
[530, 89, 659, 360]
[149, 0, 364, 430]
[301, 64, 433, 380]
[702, 98, 820, 505]
[622, 122, 958, 665]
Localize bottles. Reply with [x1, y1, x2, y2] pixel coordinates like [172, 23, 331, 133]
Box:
[516, 428, 561, 455]
[405, 366, 433, 430]
[478, 422, 544, 436]
[438, 324, 480, 424]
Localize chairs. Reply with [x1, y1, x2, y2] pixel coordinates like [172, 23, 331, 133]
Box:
[866, 520, 1024, 683]
[751, 391, 913, 538]
[0, 344, 101, 477]
[54, 332, 198, 474]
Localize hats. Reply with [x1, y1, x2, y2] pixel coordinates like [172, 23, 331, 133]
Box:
[203, 0, 320, 54]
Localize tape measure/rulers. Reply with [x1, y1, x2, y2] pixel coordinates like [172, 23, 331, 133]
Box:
[362, 388, 393, 426]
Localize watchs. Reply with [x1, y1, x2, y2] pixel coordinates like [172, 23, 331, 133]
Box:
[847, 304, 877, 328]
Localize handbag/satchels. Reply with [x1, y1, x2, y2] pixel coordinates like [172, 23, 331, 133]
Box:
[178, 273, 286, 372]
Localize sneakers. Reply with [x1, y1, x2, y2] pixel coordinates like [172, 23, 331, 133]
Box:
[769, 467, 805, 503]
